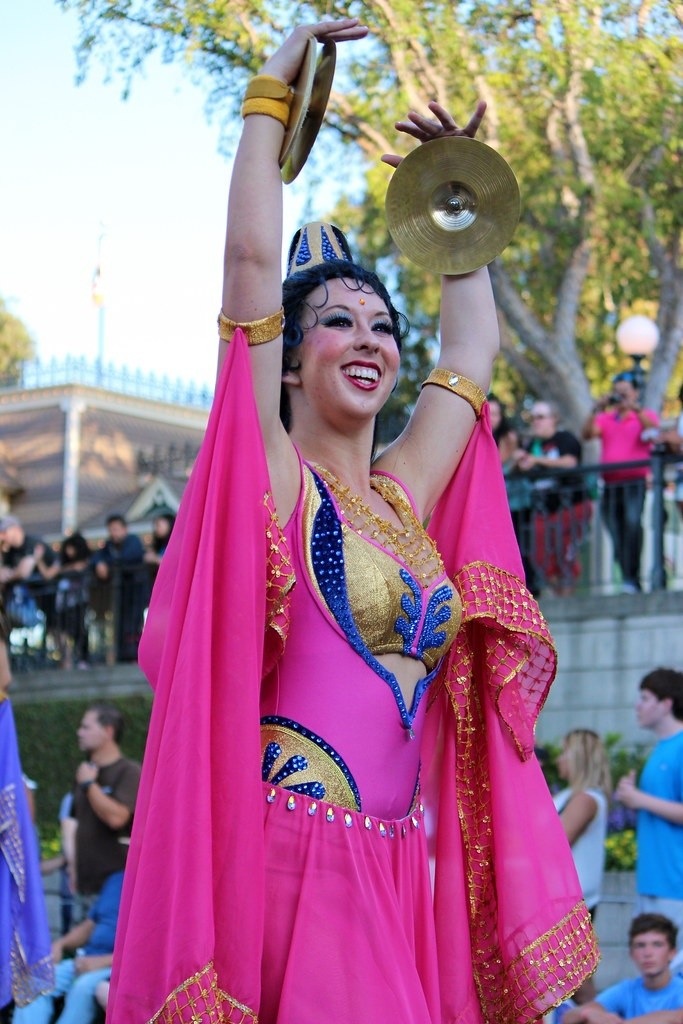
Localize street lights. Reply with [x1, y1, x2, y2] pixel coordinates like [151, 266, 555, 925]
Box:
[613, 313, 658, 406]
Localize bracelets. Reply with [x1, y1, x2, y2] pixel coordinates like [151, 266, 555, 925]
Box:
[240, 97, 291, 129]
[242, 75, 295, 108]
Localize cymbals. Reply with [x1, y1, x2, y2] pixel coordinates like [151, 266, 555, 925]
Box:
[383, 136, 521, 276]
[278, 35, 338, 186]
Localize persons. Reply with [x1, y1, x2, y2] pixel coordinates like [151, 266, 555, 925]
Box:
[561, 914, 683, 1024]
[550, 729, 612, 1005]
[60, 706, 144, 959]
[585, 368, 658, 595]
[640, 382, 682, 523]
[107, 19, 600, 1024]
[481, 392, 591, 601]
[13, 871, 125, 1024]
[0, 636, 56, 1023]
[614, 668, 682, 973]
[1, 513, 175, 669]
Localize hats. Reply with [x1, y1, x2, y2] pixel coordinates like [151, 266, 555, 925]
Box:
[0, 514, 20, 535]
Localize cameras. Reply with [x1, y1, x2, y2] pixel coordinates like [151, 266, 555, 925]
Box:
[609, 395, 622, 404]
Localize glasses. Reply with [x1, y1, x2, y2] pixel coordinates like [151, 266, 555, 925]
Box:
[527, 414, 552, 423]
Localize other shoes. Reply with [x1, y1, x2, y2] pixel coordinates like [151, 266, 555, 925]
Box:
[76, 660, 90, 671]
